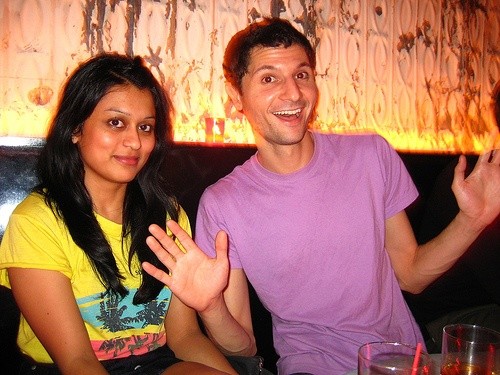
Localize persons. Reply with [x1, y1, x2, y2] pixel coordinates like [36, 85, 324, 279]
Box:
[142, 16, 500, 375]
[0, 51, 239, 375]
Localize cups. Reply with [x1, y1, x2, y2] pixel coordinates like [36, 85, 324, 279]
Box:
[440, 325, 500, 375]
[357, 342, 432, 375]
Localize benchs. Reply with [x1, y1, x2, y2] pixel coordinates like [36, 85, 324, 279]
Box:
[1, 137, 500, 375]
